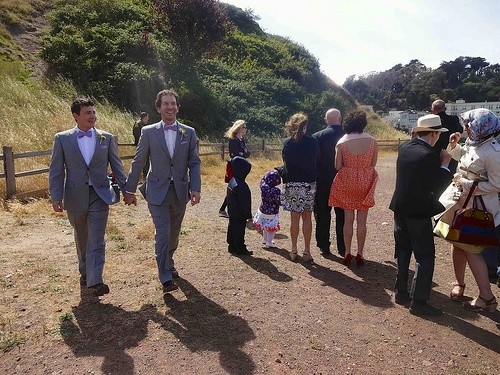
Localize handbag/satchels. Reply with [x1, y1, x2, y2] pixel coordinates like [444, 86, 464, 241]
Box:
[433, 181, 500, 253]
[225, 160, 235, 183]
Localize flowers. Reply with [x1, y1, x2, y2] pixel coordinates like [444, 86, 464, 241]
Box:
[179, 128, 185, 142]
[99, 135, 106, 144]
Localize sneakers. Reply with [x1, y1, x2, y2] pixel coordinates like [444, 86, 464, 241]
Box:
[91, 284, 109, 297]
[163, 280, 178, 293]
[219, 210, 228, 216]
[170, 267, 178, 277]
[80, 274, 87, 285]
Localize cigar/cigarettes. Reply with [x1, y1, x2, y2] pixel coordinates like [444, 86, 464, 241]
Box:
[191, 201, 195, 204]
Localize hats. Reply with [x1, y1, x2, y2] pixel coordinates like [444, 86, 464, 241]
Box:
[413, 114, 449, 133]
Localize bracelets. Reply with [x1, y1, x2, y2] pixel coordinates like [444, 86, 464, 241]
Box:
[246, 219, 253, 222]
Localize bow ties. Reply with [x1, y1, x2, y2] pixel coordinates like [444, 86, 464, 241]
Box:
[164, 124, 178, 131]
[77, 131, 92, 138]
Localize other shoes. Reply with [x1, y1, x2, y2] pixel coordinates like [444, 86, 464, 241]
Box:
[262, 240, 275, 244]
[339, 250, 345, 257]
[409, 302, 443, 316]
[394, 292, 412, 304]
[320, 247, 330, 254]
[235, 247, 253, 255]
[266, 244, 277, 248]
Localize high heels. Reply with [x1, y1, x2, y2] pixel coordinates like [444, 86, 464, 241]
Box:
[303, 251, 313, 261]
[355, 254, 364, 268]
[462, 295, 497, 313]
[343, 253, 352, 268]
[289, 252, 297, 260]
[450, 284, 465, 302]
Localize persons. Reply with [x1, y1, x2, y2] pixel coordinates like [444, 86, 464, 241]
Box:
[49, 99, 134, 296]
[219, 120, 251, 218]
[281, 112, 320, 262]
[133, 116, 150, 137]
[123, 90, 201, 295]
[252, 170, 282, 249]
[312, 108, 346, 255]
[134, 112, 150, 184]
[328, 110, 379, 269]
[411, 100, 464, 237]
[389, 114, 452, 316]
[432, 108, 500, 313]
[227, 156, 253, 257]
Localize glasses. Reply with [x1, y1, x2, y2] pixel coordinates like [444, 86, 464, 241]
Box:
[242, 127, 247, 129]
